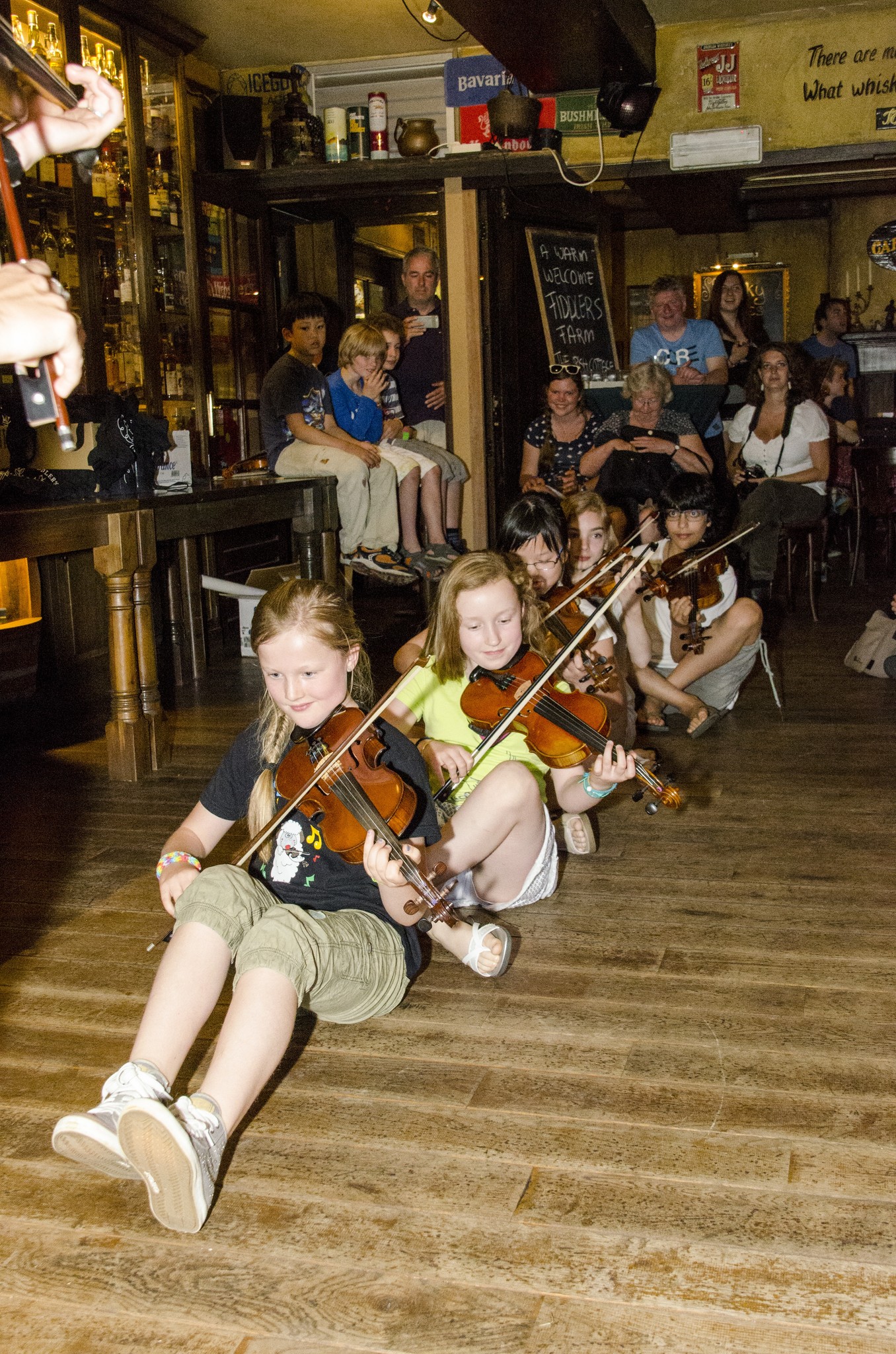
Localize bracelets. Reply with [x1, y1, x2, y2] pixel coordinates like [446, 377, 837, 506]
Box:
[157, 851, 201, 880]
[577, 772, 617, 798]
[394, 417, 403, 430]
[0, 134, 26, 188]
[370, 876, 383, 885]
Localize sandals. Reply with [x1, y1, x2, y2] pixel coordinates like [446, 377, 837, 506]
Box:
[397, 546, 445, 582]
[551, 812, 597, 856]
[424, 542, 462, 563]
[424, 905, 513, 979]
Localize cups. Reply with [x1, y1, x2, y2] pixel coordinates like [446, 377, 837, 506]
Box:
[581, 368, 631, 382]
[649, 356, 665, 366]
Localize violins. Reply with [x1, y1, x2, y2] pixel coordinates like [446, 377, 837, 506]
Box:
[660, 543, 730, 655]
[530, 576, 622, 696]
[460, 651, 687, 820]
[588, 540, 669, 601]
[272, 706, 463, 931]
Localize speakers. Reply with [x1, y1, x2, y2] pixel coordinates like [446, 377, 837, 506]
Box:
[438, 0, 657, 94]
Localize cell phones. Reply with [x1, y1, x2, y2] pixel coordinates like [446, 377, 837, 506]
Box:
[412, 315, 439, 328]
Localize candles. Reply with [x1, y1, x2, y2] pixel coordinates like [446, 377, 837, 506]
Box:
[856, 265, 860, 292]
[868, 258, 872, 286]
[846, 270, 850, 297]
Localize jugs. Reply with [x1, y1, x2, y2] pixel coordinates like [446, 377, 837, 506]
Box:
[394, 118, 440, 157]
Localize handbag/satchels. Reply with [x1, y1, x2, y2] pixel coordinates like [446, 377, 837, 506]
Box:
[845, 610, 896, 679]
[593, 426, 679, 508]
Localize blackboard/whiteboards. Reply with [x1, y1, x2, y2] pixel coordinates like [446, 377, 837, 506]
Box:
[525, 226, 622, 375]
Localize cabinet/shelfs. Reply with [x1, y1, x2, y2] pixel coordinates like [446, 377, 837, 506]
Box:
[0, 0, 298, 726]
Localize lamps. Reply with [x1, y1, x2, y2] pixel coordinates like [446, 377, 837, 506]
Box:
[594, 76, 662, 137]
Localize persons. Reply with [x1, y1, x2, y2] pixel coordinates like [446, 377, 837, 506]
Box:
[0, 63, 127, 400]
[54, 576, 445, 1234]
[258, 266, 853, 982]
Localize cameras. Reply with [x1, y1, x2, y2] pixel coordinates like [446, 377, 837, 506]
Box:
[737, 464, 768, 501]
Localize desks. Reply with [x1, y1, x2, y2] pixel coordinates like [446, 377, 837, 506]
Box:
[0, 473, 342, 783]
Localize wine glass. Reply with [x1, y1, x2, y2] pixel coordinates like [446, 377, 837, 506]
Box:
[735, 336, 747, 363]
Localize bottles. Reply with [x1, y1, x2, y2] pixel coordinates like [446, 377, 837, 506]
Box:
[98, 238, 142, 318]
[92, 146, 133, 214]
[80, 34, 126, 142]
[0, 202, 81, 309]
[103, 319, 145, 399]
[20, 154, 75, 195]
[156, 322, 195, 402]
[11, 9, 66, 83]
[147, 150, 182, 228]
[153, 234, 183, 314]
[140, 64, 177, 147]
[165, 404, 240, 476]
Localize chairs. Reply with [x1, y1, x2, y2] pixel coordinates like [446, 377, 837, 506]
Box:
[778, 519, 827, 624]
[832, 444, 857, 489]
[851, 464, 896, 587]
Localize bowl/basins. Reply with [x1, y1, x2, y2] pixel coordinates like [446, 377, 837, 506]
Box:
[531, 128, 563, 155]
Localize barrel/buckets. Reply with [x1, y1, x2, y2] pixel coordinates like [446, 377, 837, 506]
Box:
[487, 71, 542, 145]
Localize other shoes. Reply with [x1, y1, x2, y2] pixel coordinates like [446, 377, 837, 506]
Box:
[747, 587, 774, 636]
[452, 539, 472, 556]
[828, 549, 842, 557]
[627, 746, 664, 774]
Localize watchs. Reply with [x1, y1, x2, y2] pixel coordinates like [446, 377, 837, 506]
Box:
[670, 444, 679, 458]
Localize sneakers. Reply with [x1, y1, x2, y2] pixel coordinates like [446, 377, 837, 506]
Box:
[118, 1093, 227, 1234]
[51, 1062, 173, 1180]
[340, 549, 360, 566]
[349, 544, 418, 586]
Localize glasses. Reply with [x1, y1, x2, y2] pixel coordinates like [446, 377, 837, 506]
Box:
[548, 364, 581, 375]
[509, 548, 565, 571]
[662, 509, 708, 521]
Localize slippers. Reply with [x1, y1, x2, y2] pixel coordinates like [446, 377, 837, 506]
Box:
[691, 705, 729, 739]
[635, 709, 671, 732]
